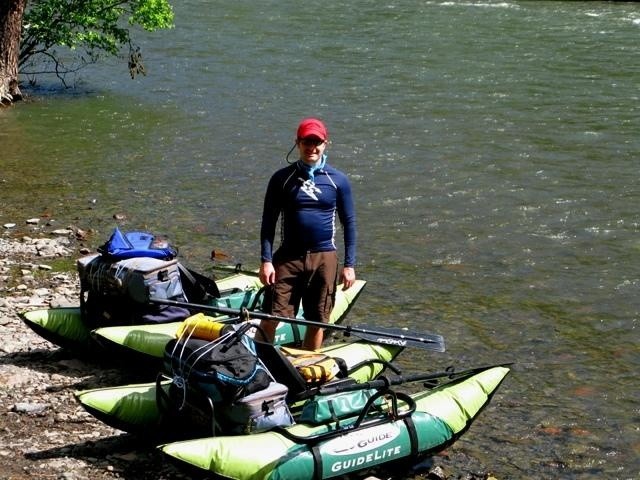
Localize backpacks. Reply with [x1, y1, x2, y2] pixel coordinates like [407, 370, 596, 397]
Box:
[163, 332, 296, 438]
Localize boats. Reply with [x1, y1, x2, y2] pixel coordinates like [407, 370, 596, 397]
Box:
[69, 326, 512, 480]
[15, 264, 367, 376]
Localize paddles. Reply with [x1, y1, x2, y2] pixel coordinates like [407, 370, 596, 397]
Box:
[149, 297, 446, 354]
[319, 362, 515, 399]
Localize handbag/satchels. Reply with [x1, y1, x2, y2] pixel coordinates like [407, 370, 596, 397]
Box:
[76, 251, 192, 326]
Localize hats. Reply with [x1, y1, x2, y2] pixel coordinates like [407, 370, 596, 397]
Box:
[296, 118, 327, 142]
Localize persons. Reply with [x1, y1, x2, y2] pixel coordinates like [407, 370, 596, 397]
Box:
[255, 118, 355, 351]
